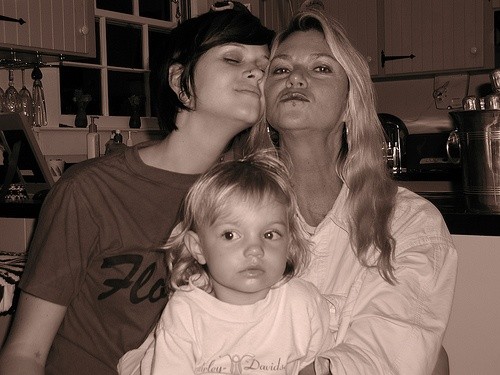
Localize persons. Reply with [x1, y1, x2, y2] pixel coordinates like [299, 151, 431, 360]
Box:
[0, 8, 276, 375]
[116, 7, 458, 375]
[140, 148, 329, 375]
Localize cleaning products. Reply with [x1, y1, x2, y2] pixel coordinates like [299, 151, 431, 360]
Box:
[110, 130, 123, 153]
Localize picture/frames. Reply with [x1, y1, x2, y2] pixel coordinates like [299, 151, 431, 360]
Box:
[0, 112, 54, 190]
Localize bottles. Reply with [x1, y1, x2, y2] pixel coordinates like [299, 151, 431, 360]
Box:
[129, 104, 141, 129]
[75, 101, 88, 128]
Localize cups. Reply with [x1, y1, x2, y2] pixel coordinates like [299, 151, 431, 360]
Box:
[31, 67, 48, 127]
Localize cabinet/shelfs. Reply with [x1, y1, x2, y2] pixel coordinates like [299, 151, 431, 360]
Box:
[0, 0, 500, 82]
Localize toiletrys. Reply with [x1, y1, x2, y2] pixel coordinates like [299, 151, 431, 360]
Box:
[88, 116, 100, 159]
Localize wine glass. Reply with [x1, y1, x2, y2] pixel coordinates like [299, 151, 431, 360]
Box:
[4, 66, 18, 112]
[17, 69, 33, 125]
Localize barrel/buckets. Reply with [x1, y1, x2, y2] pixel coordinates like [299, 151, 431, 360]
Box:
[447, 109, 500, 215]
